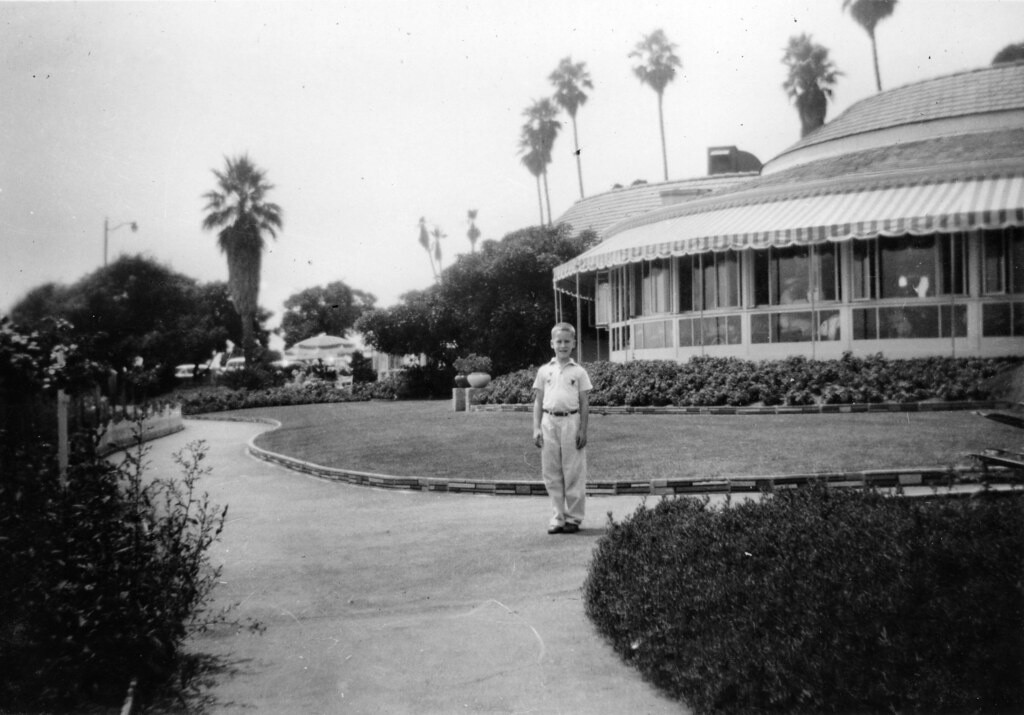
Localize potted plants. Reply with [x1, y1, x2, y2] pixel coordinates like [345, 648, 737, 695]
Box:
[452, 353, 493, 388]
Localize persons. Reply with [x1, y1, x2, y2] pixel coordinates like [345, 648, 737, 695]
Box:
[193, 364, 204, 378]
[778, 279, 814, 339]
[705, 288, 735, 338]
[305, 358, 323, 379]
[316, 357, 328, 372]
[531, 322, 593, 534]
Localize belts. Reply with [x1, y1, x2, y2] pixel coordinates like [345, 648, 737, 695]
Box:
[545, 410, 577, 417]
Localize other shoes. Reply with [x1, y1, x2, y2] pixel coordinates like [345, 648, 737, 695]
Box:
[548, 526, 565, 534]
[564, 523, 578, 534]
